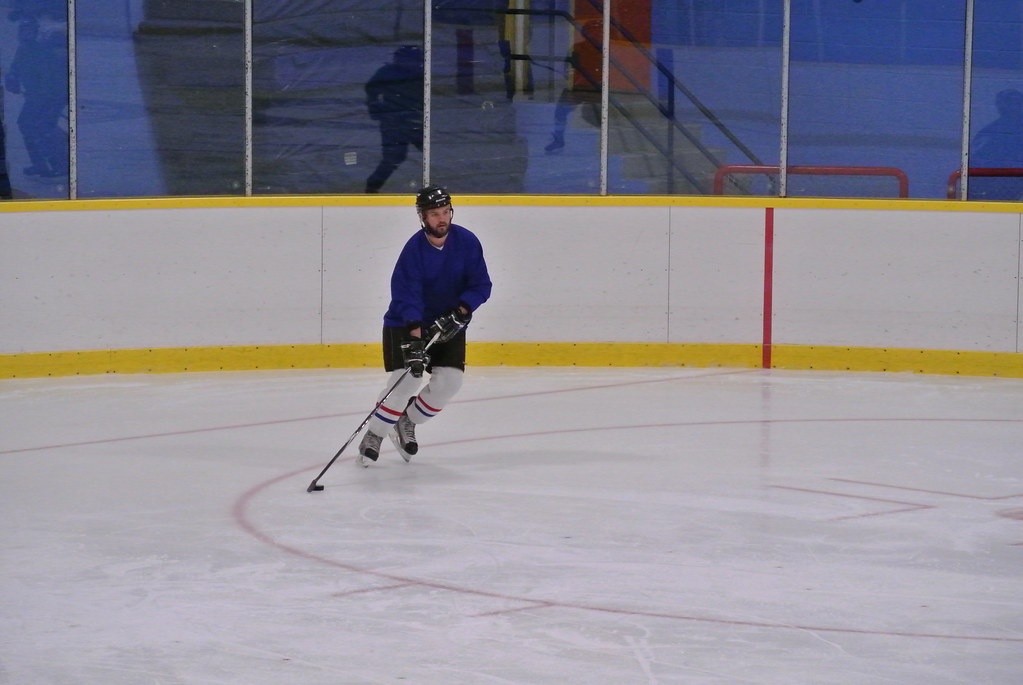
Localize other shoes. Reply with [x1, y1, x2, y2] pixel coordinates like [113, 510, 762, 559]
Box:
[365, 178, 383, 196]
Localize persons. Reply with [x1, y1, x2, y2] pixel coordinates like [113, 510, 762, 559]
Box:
[359, 184, 492, 462]
[366, 45, 424, 192]
[545, 20, 603, 152]
[971, 89, 1023, 202]
[7, 15, 68, 178]
[497, 13, 535, 98]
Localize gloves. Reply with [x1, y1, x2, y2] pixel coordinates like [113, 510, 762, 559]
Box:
[367, 96, 382, 120]
[423, 308, 471, 345]
[401, 338, 432, 377]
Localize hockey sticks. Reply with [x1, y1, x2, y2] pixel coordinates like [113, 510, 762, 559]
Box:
[306, 330, 441, 492]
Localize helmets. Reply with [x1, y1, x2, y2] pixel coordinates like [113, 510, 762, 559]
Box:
[581, 20, 603, 33]
[394, 45, 420, 62]
[416, 184, 450, 211]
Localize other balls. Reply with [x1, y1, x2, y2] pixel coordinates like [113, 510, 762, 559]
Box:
[312, 486, 324, 490]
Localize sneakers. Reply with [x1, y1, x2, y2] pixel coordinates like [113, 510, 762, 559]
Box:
[389, 396, 418, 462]
[545, 130, 565, 155]
[358, 429, 383, 468]
